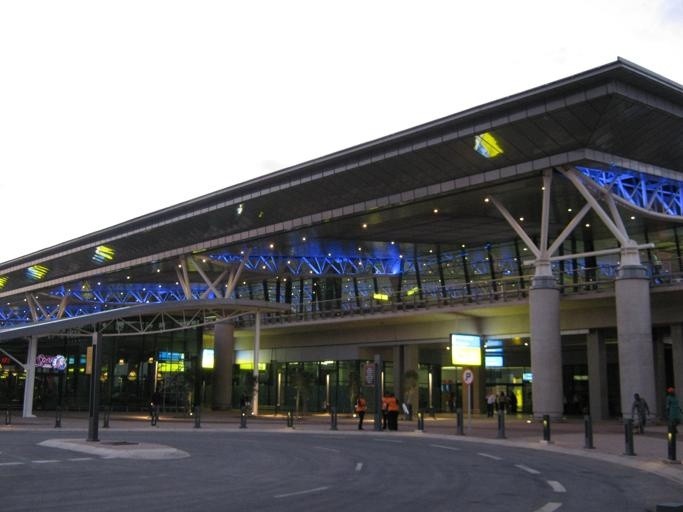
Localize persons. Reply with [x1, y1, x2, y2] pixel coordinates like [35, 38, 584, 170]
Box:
[381, 392, 389, 429]
[387, 392, 400, 431]
[666, 387, 683, 434]
[357, 393, 368, 430]
[486, 389, 519, 419]
[406, 383, 417, 422]
[240, 390, 249, 419]
[632, 394, 650, 435]
[405, 370, 419, 392]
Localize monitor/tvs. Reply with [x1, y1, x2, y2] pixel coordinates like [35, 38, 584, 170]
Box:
[449, 333, 483, 367]
